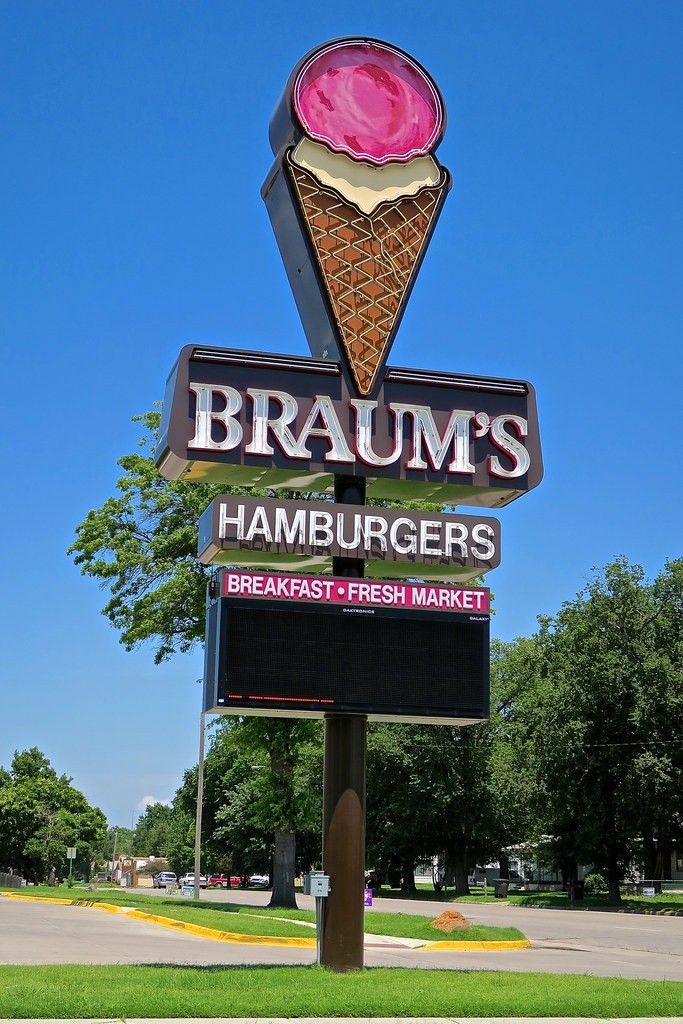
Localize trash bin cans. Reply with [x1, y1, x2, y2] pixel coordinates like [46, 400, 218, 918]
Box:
[568, 880, 585, 900]
[492, 879, 510, 898]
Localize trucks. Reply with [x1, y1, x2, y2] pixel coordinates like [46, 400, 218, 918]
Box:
[474, 866, 530, 885]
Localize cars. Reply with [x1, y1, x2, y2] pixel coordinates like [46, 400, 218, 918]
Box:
[175, 872, 207, 889]
[249, 873, 269, 887]
[153, 871, 177, 889]
[208, 872, 250, 889]
